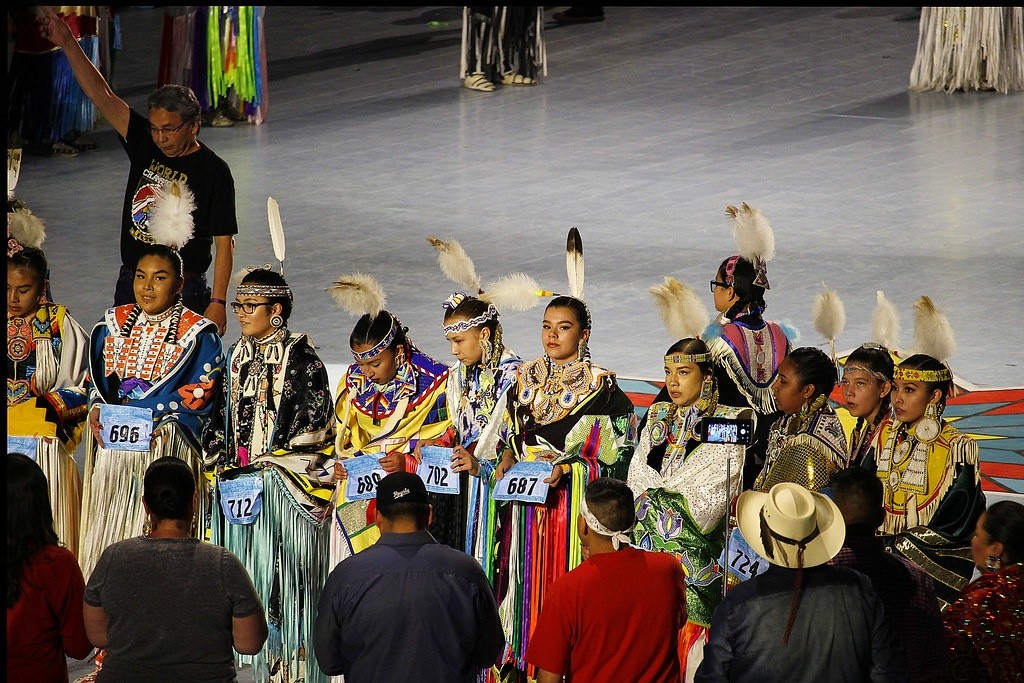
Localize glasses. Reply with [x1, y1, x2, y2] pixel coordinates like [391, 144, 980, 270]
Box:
[231, 302, 271, 313]
[710, 280, 728, 292]
[145, 119, 186, 134]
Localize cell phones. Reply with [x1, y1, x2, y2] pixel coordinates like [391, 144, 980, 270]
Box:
[701, 417, 753, 445]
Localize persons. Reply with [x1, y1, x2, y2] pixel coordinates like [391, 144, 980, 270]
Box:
[492, 295, 636, 683]
[694, 343, 1024, 683]
[157, 6, 269, 127]
[36, 6, 238, 337]
[82, 455, 270, 683]
[76, 246, 223, 683]
[6, 453, 94, 683]
[312, 470, 507, 683]
[414, 291, 526, 683]
[908, 7, 1024, 95]
[201, 266, 332, 683]
[331, 310, 453, 683]
[523, 476, 689, 683]
[460, 6, 548, 92]
[626, 337, 754, 683]
[6, 239, 91, 556]
[637, 254, 792, 486]
[36, 6, 122, 157]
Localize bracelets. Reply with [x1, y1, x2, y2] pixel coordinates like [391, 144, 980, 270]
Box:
[210, 297, 226, 306]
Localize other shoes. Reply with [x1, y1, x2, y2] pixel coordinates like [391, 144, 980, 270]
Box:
[51, 143, 79, 156]
[501, 73, 539, 86]
[220, 107, 248, 123]
[463, 74, 498, 92]
[552, 6, 605, 23]
[74, 139, 101, 153]
[201, 111, 234, 128]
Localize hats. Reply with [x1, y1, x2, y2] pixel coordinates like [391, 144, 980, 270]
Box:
[375, 471, 429, 508]
[736, 482, 846, 568]
